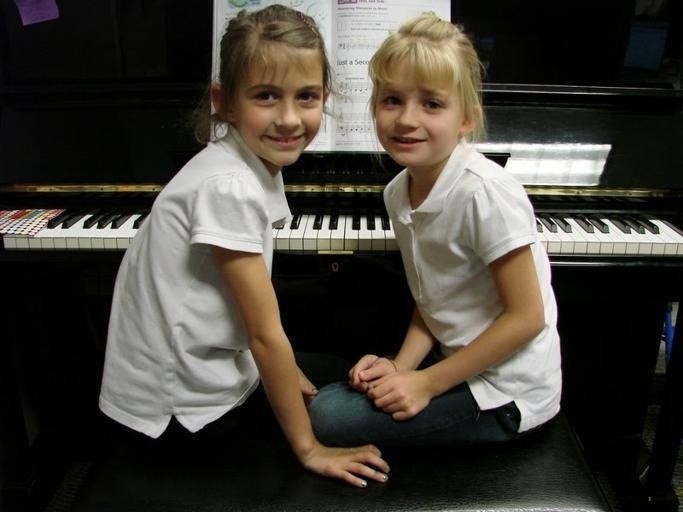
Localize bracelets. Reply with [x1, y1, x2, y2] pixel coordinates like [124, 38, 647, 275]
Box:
[390, 360, 397, 372]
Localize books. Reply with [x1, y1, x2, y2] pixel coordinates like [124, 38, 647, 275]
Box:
[211, 0, 451, 154]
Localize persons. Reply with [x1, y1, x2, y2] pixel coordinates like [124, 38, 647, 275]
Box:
[97, 4, 390, 489]
[307, 15, 564, 447]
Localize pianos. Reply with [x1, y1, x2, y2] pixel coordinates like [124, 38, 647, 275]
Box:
[0, 82, 682, 512]
